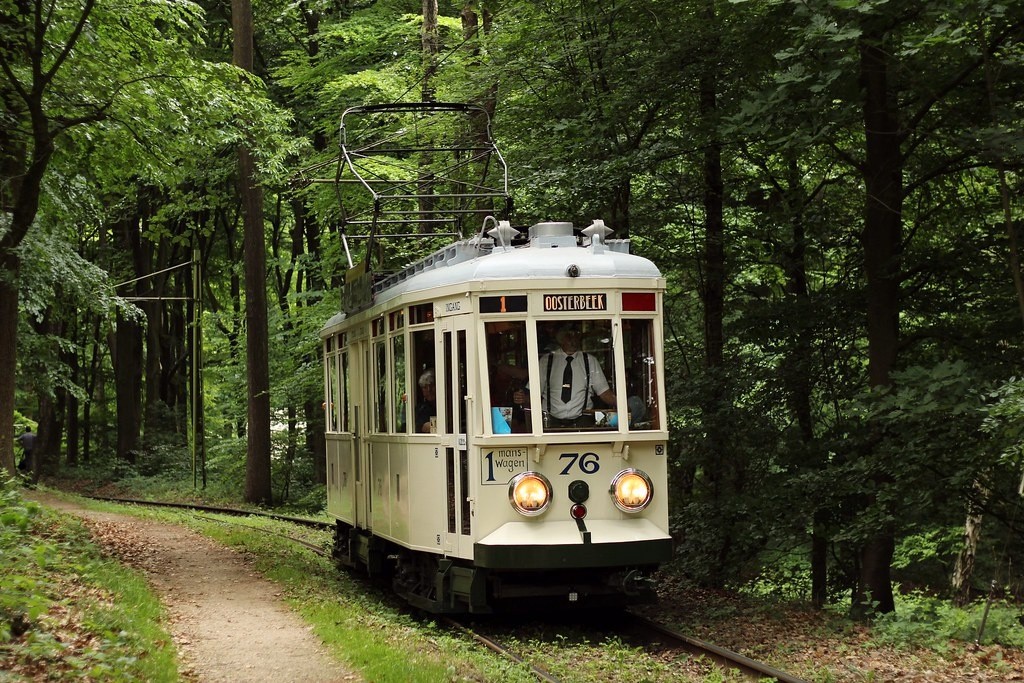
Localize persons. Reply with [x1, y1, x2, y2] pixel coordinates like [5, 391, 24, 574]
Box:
[451, 355, 528, 433]
[416, 371, 437, 433]
[512, 326, 631, 428]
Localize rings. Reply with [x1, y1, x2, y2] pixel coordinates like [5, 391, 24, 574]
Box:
[516, 399, 517, 400]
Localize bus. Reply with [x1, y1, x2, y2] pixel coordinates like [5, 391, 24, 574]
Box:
[318, 100, 675, 616]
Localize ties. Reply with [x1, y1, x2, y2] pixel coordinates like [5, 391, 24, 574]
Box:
[561, 356, 574, 403]
